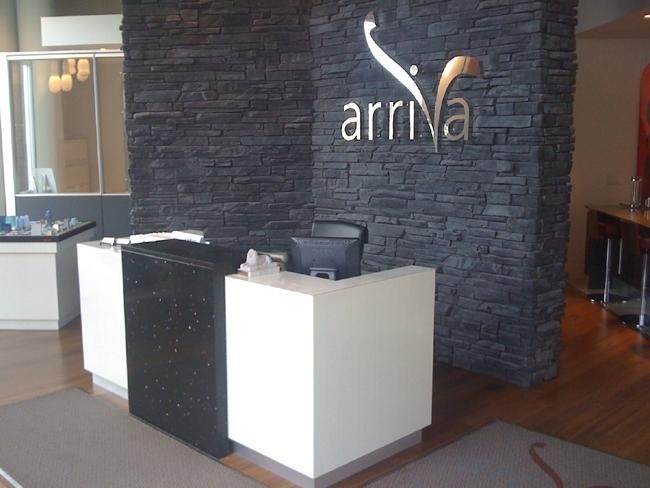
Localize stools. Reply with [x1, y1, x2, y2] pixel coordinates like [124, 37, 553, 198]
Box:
[585, 219, 628, 307]
[618, 232, 650, 338]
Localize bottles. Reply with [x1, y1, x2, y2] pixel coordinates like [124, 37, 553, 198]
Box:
[45, 209, 53, 228]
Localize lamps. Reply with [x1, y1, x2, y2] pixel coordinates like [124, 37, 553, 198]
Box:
[47, 57, 91, 93]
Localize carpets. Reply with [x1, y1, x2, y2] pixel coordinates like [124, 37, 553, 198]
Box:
[356, 416, 650, 486]
[0, 385, 271, 487]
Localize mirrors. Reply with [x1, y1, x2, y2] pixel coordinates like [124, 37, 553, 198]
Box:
[1, 51, 128, 195]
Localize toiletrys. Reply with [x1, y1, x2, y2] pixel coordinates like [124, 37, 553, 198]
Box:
[10, 209, 81, 236]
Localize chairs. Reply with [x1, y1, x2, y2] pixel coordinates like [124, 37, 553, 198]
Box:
[306, 219, 368, 256]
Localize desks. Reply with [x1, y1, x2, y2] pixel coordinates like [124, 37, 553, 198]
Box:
[584, 200, 650, 232]
